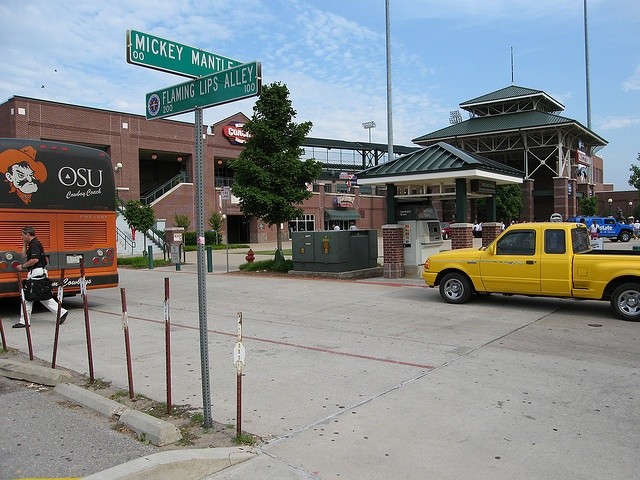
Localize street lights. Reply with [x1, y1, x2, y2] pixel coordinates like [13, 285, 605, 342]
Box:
[629, 201, 633, 215]
[607, 198, 613, 216]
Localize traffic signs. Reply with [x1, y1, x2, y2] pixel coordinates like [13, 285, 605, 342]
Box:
[146, 59, 261, 121]
[126, 29, 254, 80]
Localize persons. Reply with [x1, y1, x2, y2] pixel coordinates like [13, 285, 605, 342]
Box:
[334, 223, 341, 231]
[350, 222, 358, 230]
[11, 226, 68, 329]
[580, 218, 586, 224]
[634, 220, 640, 240]
[629, 220, 637, 238]
[472, 220, 534, 237]
[589, 220, 600, 240]
[0, 145, 47, 205]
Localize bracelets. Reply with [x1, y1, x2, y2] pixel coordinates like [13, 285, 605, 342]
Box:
[21, 264, 26, 270]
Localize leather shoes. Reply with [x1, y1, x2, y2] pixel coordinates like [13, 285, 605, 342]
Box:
[12, 323, 30, 328]
[59, 311, 69, 325]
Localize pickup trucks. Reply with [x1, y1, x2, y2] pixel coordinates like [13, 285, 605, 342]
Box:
[423, 222, 639, 321]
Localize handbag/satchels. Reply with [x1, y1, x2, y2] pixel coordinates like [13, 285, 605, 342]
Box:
[22, 277, 53, 301]
[596, 224, 600, 233]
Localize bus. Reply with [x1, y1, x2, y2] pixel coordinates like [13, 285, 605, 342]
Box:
[0, 136, 119, 298]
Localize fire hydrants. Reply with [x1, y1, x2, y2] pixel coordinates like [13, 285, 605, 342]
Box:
[245, 248, 255, 264]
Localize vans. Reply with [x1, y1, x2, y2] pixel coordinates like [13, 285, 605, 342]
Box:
[567, 216, 635, 242]
